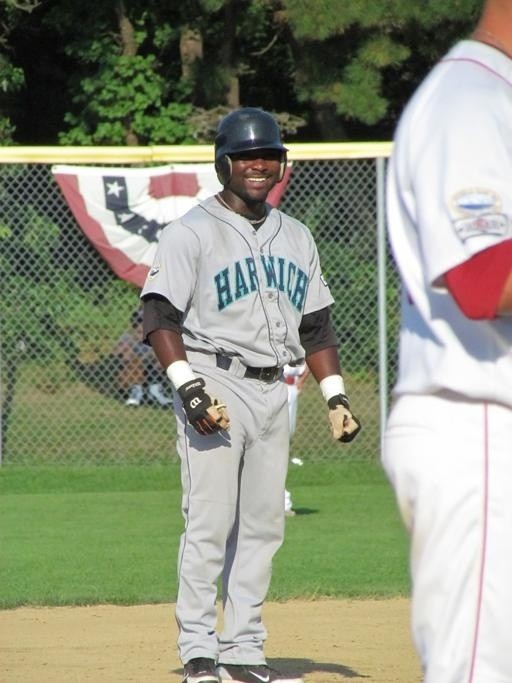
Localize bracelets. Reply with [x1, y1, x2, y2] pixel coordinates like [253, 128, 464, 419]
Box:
[166, 359, 195, 391]
[319, 375, 345, 404]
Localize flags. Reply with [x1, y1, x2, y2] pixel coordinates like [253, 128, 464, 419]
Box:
[51, 161, 293, 289]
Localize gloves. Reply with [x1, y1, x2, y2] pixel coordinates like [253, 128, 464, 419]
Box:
[324, 392, 362, 444]
[177, 378, 231, 437]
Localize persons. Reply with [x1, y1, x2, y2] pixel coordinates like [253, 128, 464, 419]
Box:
[281, 361, 311, 518]
[380, 0, 512, 682]
[113, 310, 175, 408]
[140, 109, 361, 683]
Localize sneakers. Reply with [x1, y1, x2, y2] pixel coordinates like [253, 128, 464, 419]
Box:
[218, 662, 305, 683]
[180, 658, 223, 683]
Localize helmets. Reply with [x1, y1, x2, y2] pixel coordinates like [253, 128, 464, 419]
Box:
[214, 105, 288, 157]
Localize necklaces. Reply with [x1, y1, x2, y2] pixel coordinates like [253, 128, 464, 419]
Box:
[216, 193, 269, 225]
[474, 26, 506, 49]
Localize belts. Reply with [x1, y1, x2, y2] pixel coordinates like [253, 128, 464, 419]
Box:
[212, 353, 284, 385]
